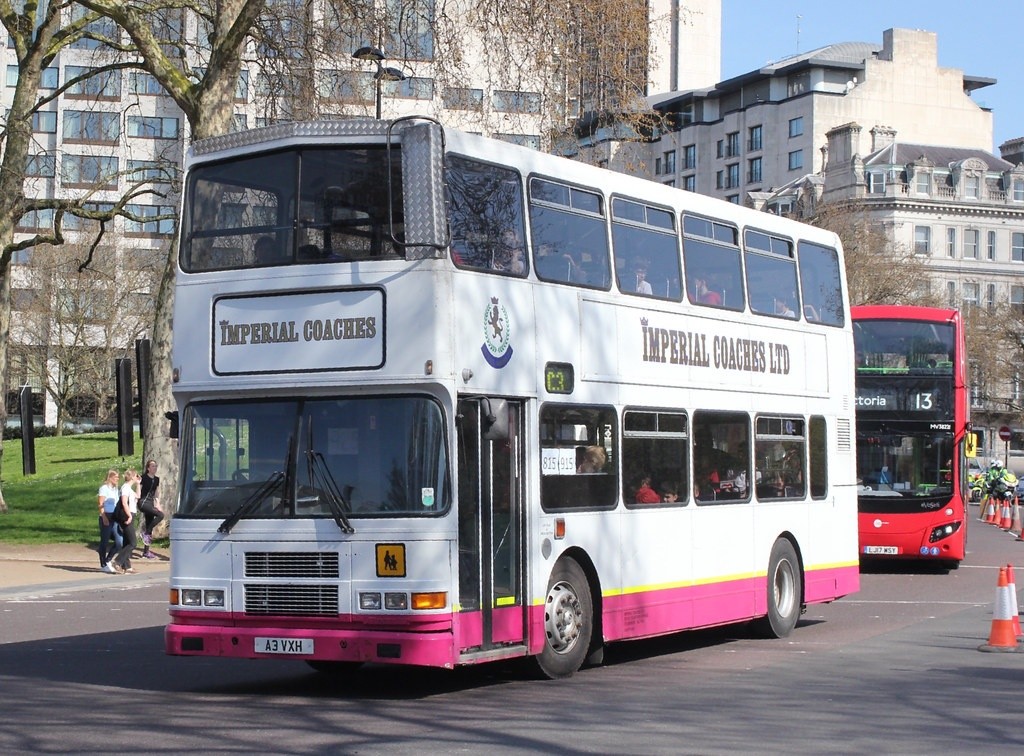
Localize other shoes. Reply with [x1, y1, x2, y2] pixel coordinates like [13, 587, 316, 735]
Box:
[124, 568, 140, 575]
[101, 562, 116, 573]
[112, 562, 123, 572]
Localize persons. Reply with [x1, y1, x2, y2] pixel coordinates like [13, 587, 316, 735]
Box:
[562, 249, 603, 288]
[567, 445, 607, 506]
[633, 265, 654, 295]
[625, 468, 678, 504]
[772, 294, 796, 319]
[985, 460, 1006, 500]
[693, 276, 721, 306]
[758, 434, 804, 497]
[98, 468, 142, 575]
[695, 428, 747, 501]
[137, 460, 164, 560]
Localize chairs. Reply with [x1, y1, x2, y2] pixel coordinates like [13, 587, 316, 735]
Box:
[910, 360, 952, 374]
[291, 236, 822, 323]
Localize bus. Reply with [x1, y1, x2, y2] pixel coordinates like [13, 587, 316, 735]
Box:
[165, 117, 859, 678]
[851, 304, 977, 559]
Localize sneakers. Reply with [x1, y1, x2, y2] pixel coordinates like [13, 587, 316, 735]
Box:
[141, 550, 157, 560]
[139, 531, 152, 546]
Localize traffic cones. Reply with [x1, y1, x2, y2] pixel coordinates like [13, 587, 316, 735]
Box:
[999, 501, 1012, 528]
[977, 566, 1023, 653]
[1004, 496, 1023, 533]
[1014, 520, 1024, 542]
[985, 497, 995, 523]
[989, 498, 1001, 525]
[986, 563, 1024, 642]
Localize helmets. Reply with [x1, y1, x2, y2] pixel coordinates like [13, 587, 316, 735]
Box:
[991, 460, 1004, 469]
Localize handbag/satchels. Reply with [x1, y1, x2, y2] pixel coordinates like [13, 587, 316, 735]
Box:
[111, 495, 130, 525]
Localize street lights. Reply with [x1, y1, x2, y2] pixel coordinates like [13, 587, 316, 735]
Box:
[352, 47, 406, 120]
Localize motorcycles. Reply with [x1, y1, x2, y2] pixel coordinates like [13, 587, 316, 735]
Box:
[968, 471, 987, 502]
[981, 468, 1018, 503]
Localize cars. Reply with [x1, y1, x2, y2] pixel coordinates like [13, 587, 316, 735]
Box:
[1017, 476, 1024, 502]
[968, 457, 984, 477]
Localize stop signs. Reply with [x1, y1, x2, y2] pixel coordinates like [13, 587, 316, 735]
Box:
[999, 426, 1011, 441]
